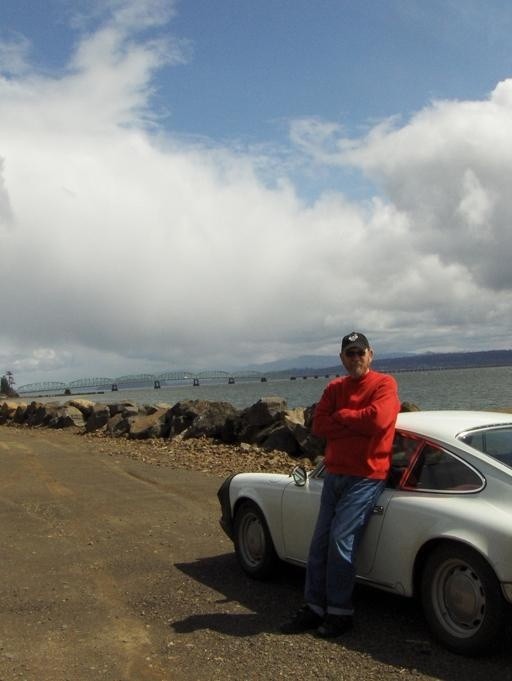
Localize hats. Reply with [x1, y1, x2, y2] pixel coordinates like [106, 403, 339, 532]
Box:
[341, 332, 371, 352]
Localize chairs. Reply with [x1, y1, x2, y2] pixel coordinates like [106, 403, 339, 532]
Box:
[427, 462, 458, 488]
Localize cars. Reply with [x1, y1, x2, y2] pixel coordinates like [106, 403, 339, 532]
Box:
[217, 409, 511, 653]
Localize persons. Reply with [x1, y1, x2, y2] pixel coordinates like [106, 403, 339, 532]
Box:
[277, 331, 400, 639]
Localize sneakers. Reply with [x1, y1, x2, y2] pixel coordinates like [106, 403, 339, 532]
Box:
[278, 603, 353, 638]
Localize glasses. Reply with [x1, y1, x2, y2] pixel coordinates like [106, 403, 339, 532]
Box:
[346, 351, 367, 357]
